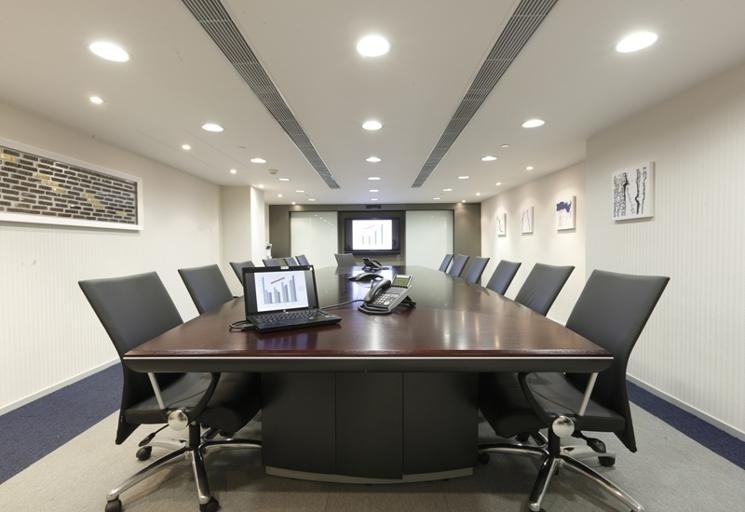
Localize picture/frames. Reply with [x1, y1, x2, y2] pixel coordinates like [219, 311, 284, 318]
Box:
[555, 194, 577, 230]
[496, 212, 507, 235]
[611, 158, 656, 221]
[520, 205, 535, 235]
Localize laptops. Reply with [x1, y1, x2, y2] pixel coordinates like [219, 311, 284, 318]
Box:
[242, 265, 342, 333]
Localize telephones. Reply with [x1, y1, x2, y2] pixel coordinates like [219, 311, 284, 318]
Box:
[361, 257, 382, 271]
[348, 273, 383, 281]
[364, 274, 414, 311]
